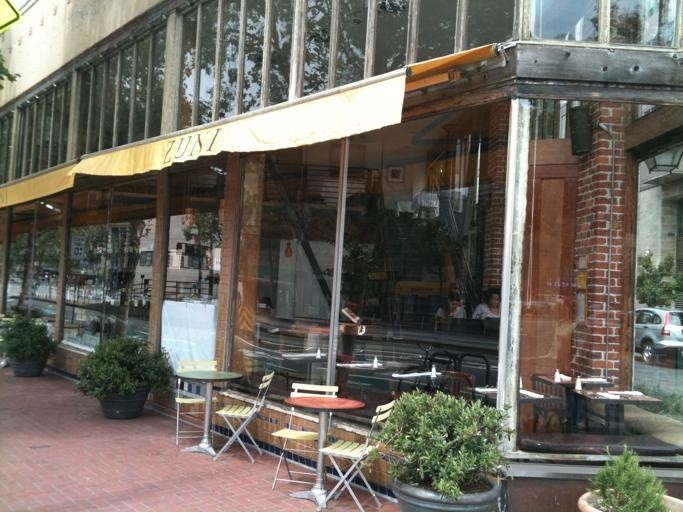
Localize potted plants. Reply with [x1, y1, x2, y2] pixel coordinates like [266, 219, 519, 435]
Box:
[4, 305, 62, 378]
[576, 440, 683, 511]
[75, 329, 174, 423]
[362, 387, 515, 512]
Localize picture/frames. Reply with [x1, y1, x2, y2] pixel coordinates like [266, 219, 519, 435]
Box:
[385, 164, 407, 184]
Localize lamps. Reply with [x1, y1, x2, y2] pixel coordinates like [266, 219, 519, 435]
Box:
[285, 232, 293, 259]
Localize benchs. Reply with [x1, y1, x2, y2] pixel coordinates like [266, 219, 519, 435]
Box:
[519, 425, 683, 457]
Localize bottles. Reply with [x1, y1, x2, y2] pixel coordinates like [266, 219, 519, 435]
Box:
[554, 369, 561, 383]
[430, 364, 437, 378]
[373, 355, 379, 369]
[574, 376, 583, 391]
[316, 346, 322, 359]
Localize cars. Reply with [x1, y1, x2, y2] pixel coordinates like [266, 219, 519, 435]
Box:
[635, 307, 683, 366]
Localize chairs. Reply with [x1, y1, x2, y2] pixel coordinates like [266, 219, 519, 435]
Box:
[174, 357, 398, 512]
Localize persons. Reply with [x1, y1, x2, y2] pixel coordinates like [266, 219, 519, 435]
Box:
[472, 286, 500, 319]
[436, 293, 467, 318]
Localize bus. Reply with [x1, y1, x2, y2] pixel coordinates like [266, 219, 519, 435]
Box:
[41, 268, 99, 297]
[99, 248, 210, 301]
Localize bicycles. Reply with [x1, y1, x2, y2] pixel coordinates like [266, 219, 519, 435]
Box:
[397, 342, 475, 403]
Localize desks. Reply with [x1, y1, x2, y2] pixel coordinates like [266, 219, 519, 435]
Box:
[461, 363, 666, 435]
